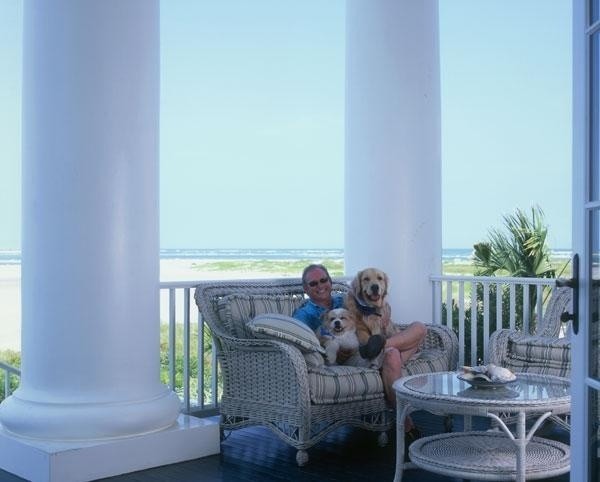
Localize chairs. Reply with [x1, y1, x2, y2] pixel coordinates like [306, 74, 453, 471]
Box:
[487, 286, 571, 435]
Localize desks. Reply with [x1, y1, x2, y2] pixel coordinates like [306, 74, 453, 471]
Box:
[391, 369, 573, 481]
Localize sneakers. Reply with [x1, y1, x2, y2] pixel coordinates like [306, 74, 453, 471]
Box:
[406, 428, 421, 447]
[359, 334, 386, 359]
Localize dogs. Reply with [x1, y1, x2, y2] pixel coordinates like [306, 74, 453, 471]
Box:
[315, 308, 359, 364]
[344, 268, 400, 345]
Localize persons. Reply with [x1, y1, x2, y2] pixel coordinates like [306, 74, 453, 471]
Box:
[290, 263, 428, 444]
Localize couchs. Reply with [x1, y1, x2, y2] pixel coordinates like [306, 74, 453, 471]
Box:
[195, 280, 459, 465]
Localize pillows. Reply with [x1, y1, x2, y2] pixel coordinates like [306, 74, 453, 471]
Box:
[245, 310, 327, 369]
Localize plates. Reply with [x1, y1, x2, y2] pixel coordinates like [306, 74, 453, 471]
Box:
[458, 373, 515, 388]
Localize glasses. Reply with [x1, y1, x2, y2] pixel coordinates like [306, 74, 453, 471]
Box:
[309, 278, 328, 286]
